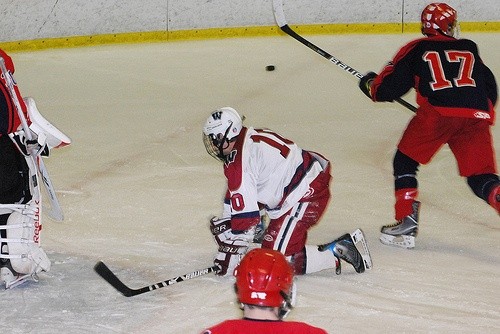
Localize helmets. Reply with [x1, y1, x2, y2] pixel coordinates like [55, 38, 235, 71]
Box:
[421, 3, 460, 36]
[233, 247, 295, 318]
[201, 107, 242, 160]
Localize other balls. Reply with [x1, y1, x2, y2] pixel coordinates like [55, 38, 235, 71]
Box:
[264, 64, 276, 72]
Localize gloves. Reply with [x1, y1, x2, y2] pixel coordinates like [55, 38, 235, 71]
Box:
[213, 237, 251, 278]
[210, 214, 236, 247]
[359, 70, 378, 100]
[25, 123, 48, 157]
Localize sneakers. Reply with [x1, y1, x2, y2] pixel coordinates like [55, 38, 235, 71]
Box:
[377, 200, 422, 248]
[317, 228, 372, 273]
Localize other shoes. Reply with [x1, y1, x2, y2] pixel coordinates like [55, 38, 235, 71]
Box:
[0, 261, 40, 290]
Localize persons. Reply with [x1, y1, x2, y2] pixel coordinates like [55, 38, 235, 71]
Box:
[200, 249, 331, 334]
[358, 2, 500, 249]
[0, 48, 51, 291]
[203, 107, 374, 278]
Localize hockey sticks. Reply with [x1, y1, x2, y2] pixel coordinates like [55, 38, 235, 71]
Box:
[92, 258, 221, 298]
[272, 0, 420, 114]
[0, 55, 68, 224]
